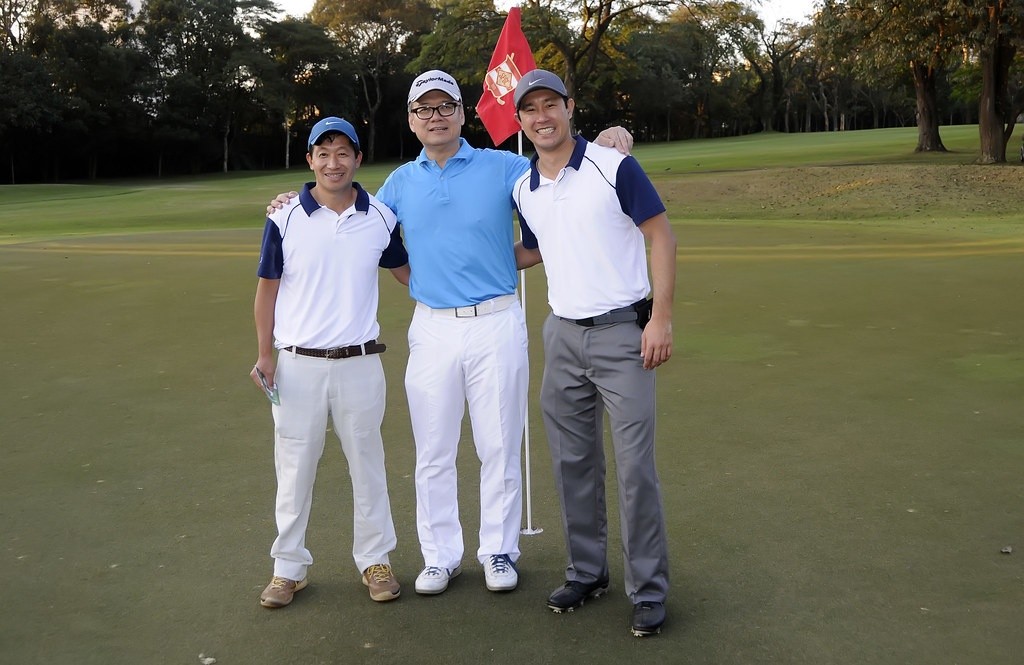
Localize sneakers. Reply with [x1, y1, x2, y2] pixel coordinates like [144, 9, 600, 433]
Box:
[260, 576, 307, 607]
[483, 554, 520, 589]
[362, 564, 400, 601]
[415, 563, 461, 594]
[547, 573, 610, 613]
[630, 599, 665, 636]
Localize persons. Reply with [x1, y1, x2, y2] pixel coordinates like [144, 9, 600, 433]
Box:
[251, 116, 411, 600]
[514, 73, 676, 635]
[266, 70, 633, 592]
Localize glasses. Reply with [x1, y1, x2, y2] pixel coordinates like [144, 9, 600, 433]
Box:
[411, 101, 461, 120]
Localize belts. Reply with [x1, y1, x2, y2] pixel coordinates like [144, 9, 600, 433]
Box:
[562, 297, 648, 327]
[415, 289, 519, 318]
[284, 339, 387, 359]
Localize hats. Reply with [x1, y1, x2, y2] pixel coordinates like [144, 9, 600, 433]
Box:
[407, 70, 462, 109]
[307, 117, 360, 156]
[514, 69, 567, 108]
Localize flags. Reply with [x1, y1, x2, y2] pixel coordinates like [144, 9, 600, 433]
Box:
[474, 7, 539, 147]
